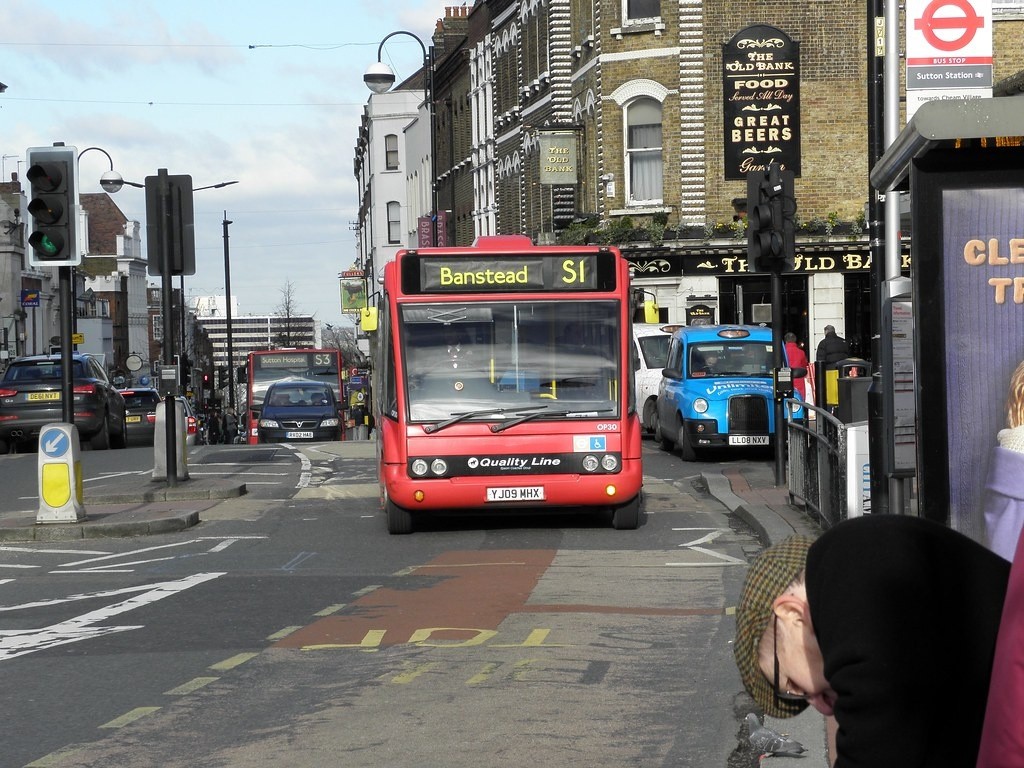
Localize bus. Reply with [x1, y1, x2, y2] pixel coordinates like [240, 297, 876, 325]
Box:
[360, 235, 644, 531]
[235, 349, 348, 444]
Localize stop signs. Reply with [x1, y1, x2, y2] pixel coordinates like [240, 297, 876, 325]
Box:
[906, 0, 993, 113]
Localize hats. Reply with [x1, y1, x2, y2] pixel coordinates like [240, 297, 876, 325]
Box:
[733, 534, 815, 720]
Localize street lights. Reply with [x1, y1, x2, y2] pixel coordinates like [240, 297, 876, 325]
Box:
[362, 28, 440, 246]
[72, 147, 124, 356]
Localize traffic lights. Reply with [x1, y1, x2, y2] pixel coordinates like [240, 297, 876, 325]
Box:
[24, 145, 82, 267]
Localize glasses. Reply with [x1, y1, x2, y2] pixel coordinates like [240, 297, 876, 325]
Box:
[773, 593, 806, 705]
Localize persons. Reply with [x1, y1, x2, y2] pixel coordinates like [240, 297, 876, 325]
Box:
[351, 403, 374, 439]
[816, 325, 852, 366]
[785, 333, 808, 402]
[694, 353, 725, 367]
[733, 510, 1024, 766]
[209, 408, 241, 445]
[439, 333, 466, 368]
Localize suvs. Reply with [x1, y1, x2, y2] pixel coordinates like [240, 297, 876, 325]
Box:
[0, 354, 130, 453]
[169, 397, 197, 446]
[115, 387, 164, 448]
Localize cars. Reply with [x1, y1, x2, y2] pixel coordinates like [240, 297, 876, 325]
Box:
[629, 321, 674, 430]
[257, 380, 342, 444]
[656, 322, 807, 463]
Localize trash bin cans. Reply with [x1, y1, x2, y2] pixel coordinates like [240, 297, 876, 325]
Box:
[152, 398, 190, 482]
[836, 357, 873, 377]
